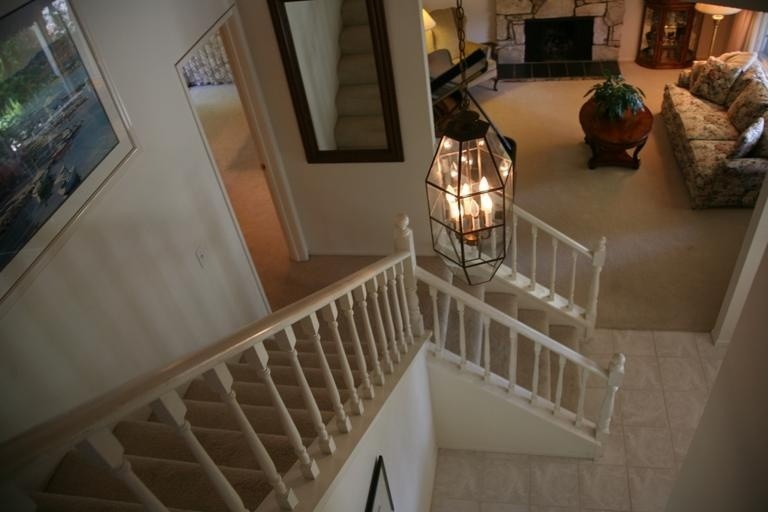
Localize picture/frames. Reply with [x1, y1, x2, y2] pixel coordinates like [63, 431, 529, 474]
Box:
[0, 0, 144, 318]
[364, 455, 396, 512]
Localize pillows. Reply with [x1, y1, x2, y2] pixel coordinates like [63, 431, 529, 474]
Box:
[678, 70, 692, 88]
[723, 68, 757, 109]
[689, 55, 741, 106]
[746, 109, 768, 161]
[726, 117, 765, 159]
[727, 79, 768, 132]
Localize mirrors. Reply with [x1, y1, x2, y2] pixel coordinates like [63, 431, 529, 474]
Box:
[265, 0, 405, 163]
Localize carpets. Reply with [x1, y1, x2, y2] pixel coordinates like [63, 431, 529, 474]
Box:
[454, 68, 760, 332]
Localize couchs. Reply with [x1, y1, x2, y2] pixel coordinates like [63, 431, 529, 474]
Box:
[422, 6, 497, 107]
[662, 51, 768, 210]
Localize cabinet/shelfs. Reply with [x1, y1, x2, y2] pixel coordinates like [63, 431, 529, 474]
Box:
[635, 0, 694, 69]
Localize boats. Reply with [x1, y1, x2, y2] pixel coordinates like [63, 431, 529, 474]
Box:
[54, 164, 77, 196]
[23, 158, 49, 198]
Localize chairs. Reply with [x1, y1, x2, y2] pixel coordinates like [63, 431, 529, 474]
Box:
[428, 49, 456, 80]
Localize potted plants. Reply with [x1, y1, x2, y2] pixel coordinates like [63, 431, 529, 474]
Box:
[581, 70, 647, 123]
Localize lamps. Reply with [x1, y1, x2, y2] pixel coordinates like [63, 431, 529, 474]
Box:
[425, 0, 514, 286]
[694, 3, 742, 60]
[422, 7, 437, 31]
